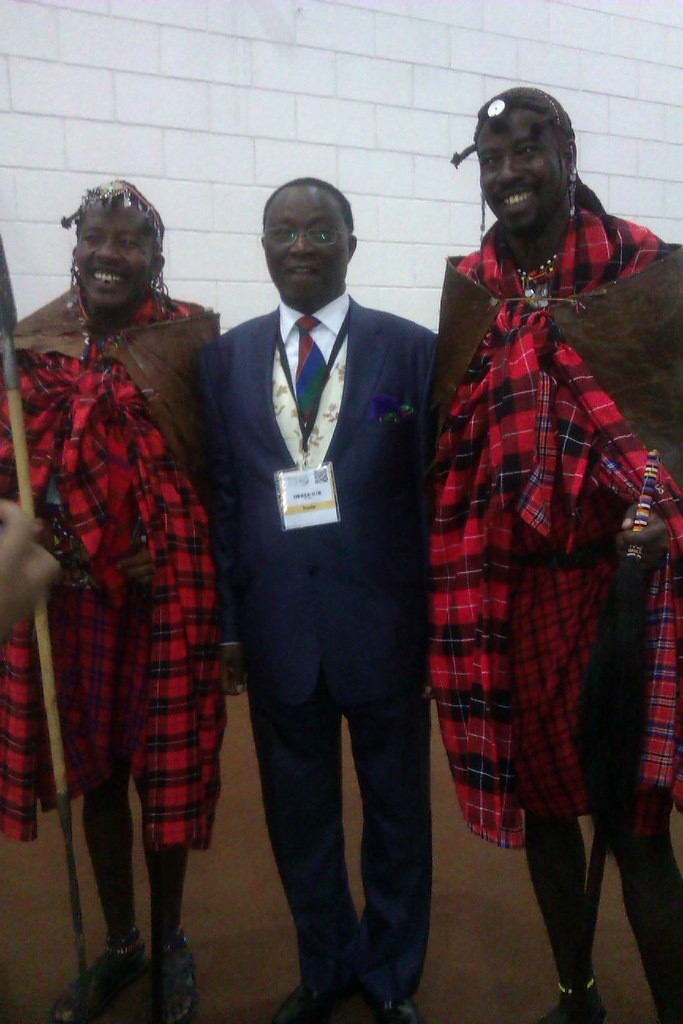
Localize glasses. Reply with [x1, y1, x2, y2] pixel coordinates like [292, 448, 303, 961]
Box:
[265, 223, 350, 249]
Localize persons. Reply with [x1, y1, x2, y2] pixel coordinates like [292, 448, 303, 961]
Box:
[196, 180, 442, 1024]
[420, 87, 683, 1024]
[0, 177, 220, 1024]
[0, 498, 62, 632]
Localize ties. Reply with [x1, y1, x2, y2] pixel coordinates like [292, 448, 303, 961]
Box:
[294, 315, 330, 428]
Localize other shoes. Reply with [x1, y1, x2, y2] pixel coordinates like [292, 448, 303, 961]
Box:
[375, 998, 425, 1023]
[536, 1003, 607, 1024]
[272, 982, 330, 1024]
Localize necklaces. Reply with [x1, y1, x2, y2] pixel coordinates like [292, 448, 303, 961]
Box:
[513, 255, 557, 309]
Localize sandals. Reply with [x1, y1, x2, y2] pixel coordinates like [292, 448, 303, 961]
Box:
[48, 940, 148, 1024]
[155, 933, 196, 1024]
